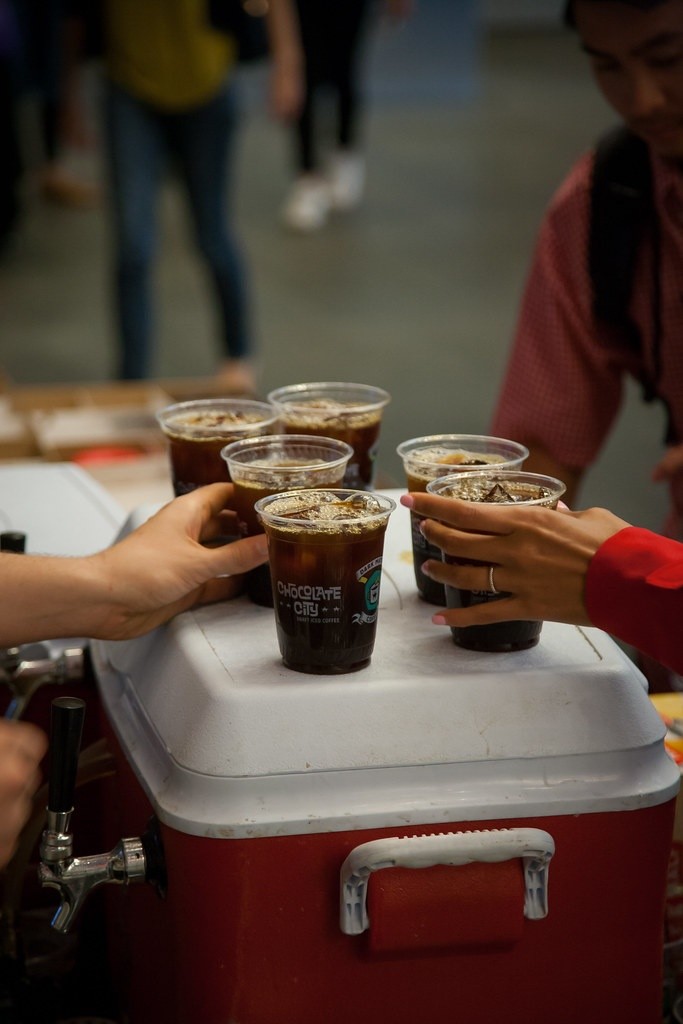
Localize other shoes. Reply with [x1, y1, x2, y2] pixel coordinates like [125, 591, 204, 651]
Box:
[279, 149, 366, 234]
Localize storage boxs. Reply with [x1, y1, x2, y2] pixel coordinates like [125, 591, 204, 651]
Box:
[84, 485, 683, 1024]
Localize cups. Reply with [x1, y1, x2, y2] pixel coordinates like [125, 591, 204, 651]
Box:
[396, 432, 530, 607]
[427, 470, 566, 652]
[255, 487, 396, 677]
[219, 433, 355, 607]
[157, 399, 278, 501]
[268, 380, 389, 493]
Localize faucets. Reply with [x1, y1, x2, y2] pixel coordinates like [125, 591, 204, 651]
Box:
[29, 693, 168, 937]
[0, 528, 102, 731]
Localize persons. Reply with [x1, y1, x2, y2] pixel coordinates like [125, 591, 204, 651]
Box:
[492, 3, 683, 508]
[1, 481, 273, 647]
[286, 0, 370, 230]
[400, 488, 683, 679]
[100, 3, 276, 392]
